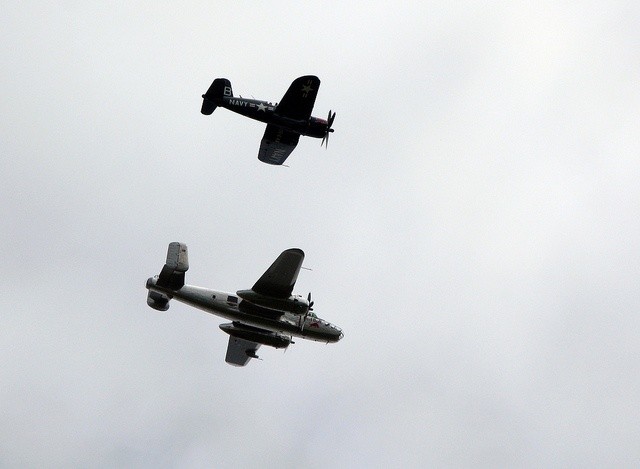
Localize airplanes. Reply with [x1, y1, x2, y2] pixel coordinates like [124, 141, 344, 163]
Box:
[146, 242, 344, 367]
[200, 75, 336, 165]
